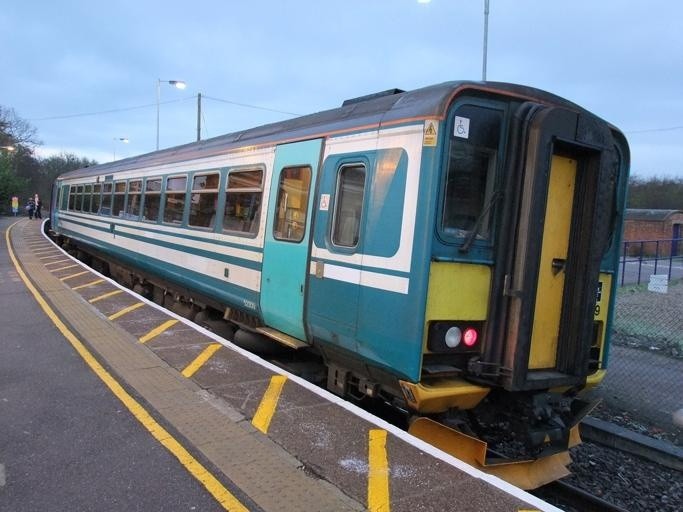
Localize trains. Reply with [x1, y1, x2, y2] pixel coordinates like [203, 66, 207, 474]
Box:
[49, 79, 631, 492]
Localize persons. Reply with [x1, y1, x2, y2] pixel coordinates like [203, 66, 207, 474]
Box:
[33, 193, 42, 220]
[26, 197, 35, 219]
